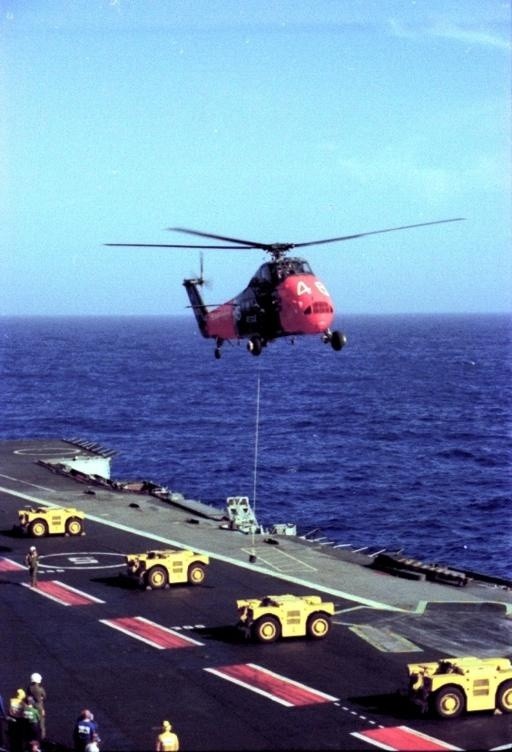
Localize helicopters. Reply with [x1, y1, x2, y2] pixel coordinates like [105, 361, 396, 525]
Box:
[105, 218, 466, 359]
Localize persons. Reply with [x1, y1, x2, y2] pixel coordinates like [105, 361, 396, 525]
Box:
[3, 688, 27, 738]
[24, 544, 39, 589]
[26, 672, 46, 737]
[155, 721, 180, 752]
[75, 707, 103, 752]
[17, 697, 46, 752]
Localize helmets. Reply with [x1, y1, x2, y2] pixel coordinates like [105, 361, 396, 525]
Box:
[30, 672, 42, 684]
